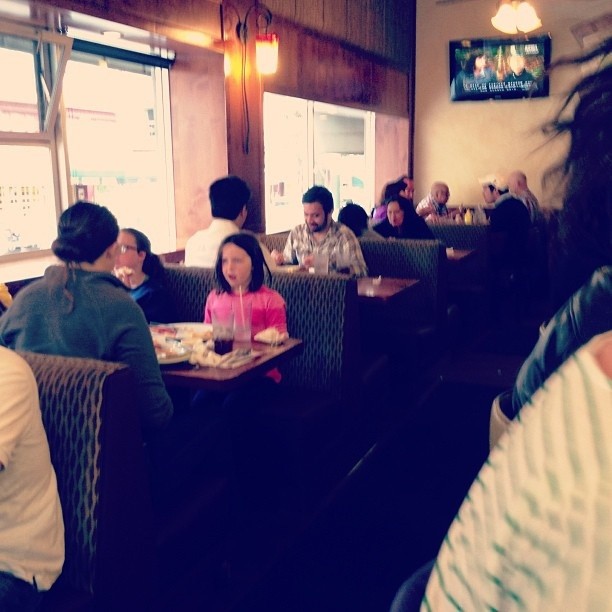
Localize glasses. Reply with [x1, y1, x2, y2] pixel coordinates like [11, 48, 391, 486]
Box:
[488, 0, 543, 35]
[242, 3, 279, 75]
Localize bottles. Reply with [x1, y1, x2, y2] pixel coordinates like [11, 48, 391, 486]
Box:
[477, 173, 509, 192]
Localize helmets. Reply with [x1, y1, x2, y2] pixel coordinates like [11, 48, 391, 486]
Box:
[149, 322, 214, 364]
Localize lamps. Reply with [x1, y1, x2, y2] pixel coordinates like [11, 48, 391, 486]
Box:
[158, 264, 348, 394]
[252, 234, 439, 324]
[366, 216, 489, 249]
[12, 349, 136, 601]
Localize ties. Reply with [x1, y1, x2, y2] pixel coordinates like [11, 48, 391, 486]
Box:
[449, 32, 551, 101]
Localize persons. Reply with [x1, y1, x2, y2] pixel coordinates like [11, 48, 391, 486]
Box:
[271, 185, 369, 281]
[421, 326, 612, 612]
[2, 201, 173, 452]
[502, 33, 612, 408]
[416, 180, 473, 260]
[183, 175, 277, 269]
[1, 340, 68, 611]
[372, 179, 407, 224]
[110, 226, 174, 326]
[455, 51, 497, 92]
[204, 234, 295, 428]
[400, 175, 415, 212]
[372, 199, 435, 244]
[506, 170, 541, 227]
[480, 173, 532, 281]
[503, 47, 535, 87]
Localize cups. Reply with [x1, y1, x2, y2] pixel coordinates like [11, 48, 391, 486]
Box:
[118, 242, 135, 253]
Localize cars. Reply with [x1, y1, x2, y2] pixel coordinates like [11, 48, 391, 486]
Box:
[234, 296, 253, 355]
[312, 254, 328, 276]
[212, 305, 233, 356]
